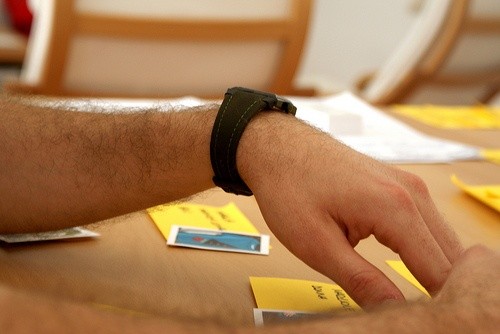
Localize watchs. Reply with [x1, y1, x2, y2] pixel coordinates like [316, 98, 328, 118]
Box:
[210, 87, 298, 195]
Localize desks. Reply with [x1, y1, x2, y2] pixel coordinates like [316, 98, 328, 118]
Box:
[1, 93, 500, 333]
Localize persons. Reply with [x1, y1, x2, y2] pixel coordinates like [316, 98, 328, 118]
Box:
[0, 86, 500, 334]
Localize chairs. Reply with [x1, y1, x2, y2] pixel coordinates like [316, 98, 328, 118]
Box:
[3, 0, 500, 105]
